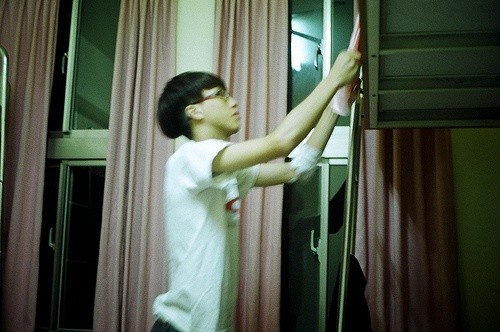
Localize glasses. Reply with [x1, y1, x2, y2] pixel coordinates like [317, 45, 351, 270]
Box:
[190, 89, 229, 106]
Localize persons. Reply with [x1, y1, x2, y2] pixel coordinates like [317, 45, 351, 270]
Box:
[281, 180, 347, 332]
[150, 50, 362, 332]
